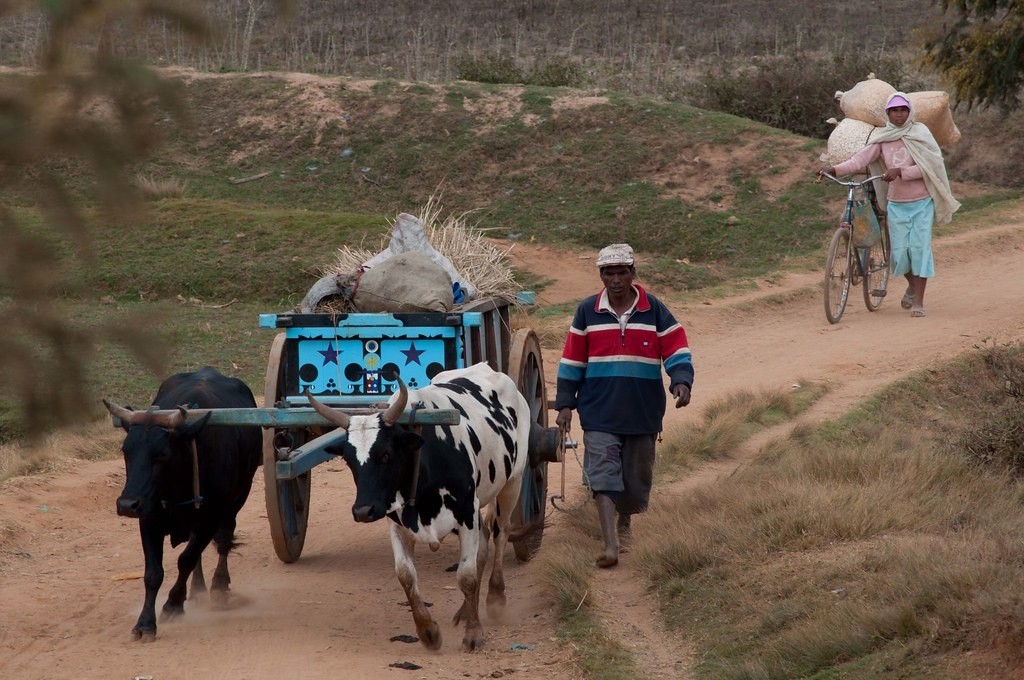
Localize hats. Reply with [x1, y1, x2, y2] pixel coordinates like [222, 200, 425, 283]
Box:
[885, 96, 910, 115]
[595, 243, 634, 268]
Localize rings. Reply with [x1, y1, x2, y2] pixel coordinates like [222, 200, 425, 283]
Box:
[883, 173, 886, 176]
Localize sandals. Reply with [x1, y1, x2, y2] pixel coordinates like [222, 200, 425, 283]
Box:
[910, 306, 926, 317]
[900, 288, 916, 309]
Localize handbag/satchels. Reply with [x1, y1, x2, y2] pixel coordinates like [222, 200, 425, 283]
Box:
[852, 182, 882, 248]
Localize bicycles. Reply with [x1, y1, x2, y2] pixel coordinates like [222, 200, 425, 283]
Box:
[809, 168, 897, 324]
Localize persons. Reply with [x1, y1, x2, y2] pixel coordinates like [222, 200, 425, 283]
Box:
[552, 243, 695, 568]
[810, 91, 944, 318]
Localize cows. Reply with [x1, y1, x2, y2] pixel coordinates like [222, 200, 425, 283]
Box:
[305, 361, 533, 655]
[102, 365, 265, 643]
